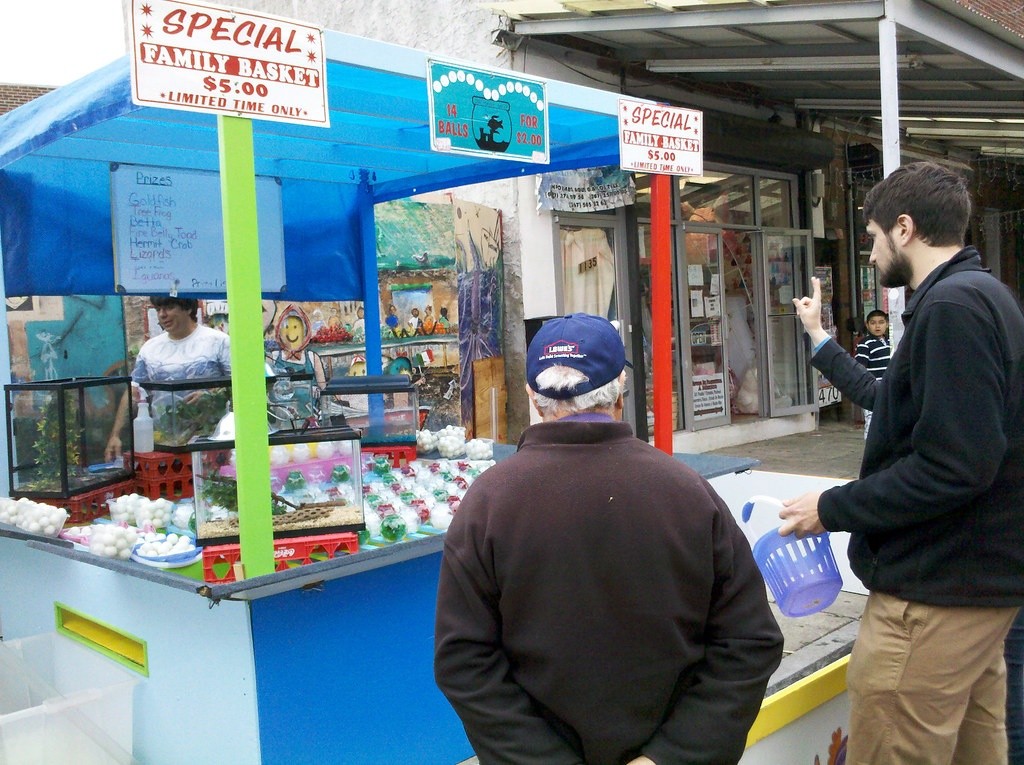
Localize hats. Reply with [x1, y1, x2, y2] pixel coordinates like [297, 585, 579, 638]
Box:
[526, 312, 634, 400]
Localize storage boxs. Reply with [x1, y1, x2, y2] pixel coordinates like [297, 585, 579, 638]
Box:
[186, 425, 366, 547]
[139, 378, 235, 456]
[320, 374, 417, 446]
[266, 372, 315, 429]
[0, 631, 139, 765]
[123, 450, 230, 482]
[4, 376, 136, 499]
[361, 446, 416, 468]
[15, 480, 135, 523]
[202, 532, 359, 584]
[134, 474, 195, 500]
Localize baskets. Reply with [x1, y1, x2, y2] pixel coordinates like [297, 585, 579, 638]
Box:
[753, 525, 842, 617]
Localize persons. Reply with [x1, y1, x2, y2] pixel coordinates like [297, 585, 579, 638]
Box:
[105, 295, 232, 462]
[435, 313, 783, 765]
[313, 303, 451, 335]
[780, 162, 1023, 764]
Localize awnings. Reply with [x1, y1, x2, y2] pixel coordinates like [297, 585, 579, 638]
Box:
[508, 1, 1024, 117]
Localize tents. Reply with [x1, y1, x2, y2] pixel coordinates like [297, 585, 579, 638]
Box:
[0, 26, 670, 579]
